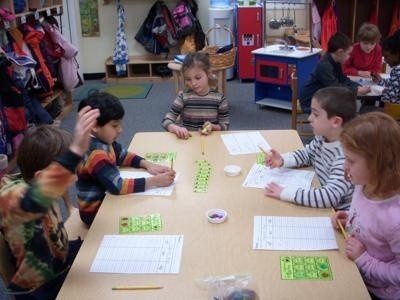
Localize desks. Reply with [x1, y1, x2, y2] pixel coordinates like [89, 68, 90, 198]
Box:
[54, 129, 373, 300]
[347, 73, 390, 105]
[168, 61, 234, 97]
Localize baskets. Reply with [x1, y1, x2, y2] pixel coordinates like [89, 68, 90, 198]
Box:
[201, 25, 238, 70]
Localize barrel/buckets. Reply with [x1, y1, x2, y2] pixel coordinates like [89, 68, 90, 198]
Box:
[209, 0, 230, 8]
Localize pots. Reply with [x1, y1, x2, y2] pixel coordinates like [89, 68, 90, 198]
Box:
[269, 1, 294, 29]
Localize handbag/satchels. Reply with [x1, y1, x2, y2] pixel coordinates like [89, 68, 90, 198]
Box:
[7, 51, 39, 89]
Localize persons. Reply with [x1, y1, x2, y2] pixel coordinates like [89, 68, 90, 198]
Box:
[263, 23, 400, 300]
[74, 88, 176, 224]
[160, 52, 230, 140]
[0, 105, 101, 300]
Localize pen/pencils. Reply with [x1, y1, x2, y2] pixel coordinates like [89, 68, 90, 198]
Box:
[112, 286, 164, 290]
[171, 156, 173, 172]
[331, 207, 348, 239]
[201, 137, 205, 156]
[256, 144, 280, 168]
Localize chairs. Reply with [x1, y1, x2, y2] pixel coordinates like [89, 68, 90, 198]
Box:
[50, 187, 90, 241]
[291, 65, 315, 137]
[383, 101, 400, 122]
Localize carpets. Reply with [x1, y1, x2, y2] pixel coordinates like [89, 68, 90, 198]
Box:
[73, 83, 154, 99]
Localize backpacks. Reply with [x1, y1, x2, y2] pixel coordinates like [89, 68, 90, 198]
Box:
[151, 12, 178, 50]
[172, 3, 198, 36]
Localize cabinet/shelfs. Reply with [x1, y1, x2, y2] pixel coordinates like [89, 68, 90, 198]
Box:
[0, 0, 73, 127]
[105, 55, 181, 82]
[254, 54, 321, 114]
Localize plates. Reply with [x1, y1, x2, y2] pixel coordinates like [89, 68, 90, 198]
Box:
[206, 208, 227, 224]
[224, 165, 241, 176]
